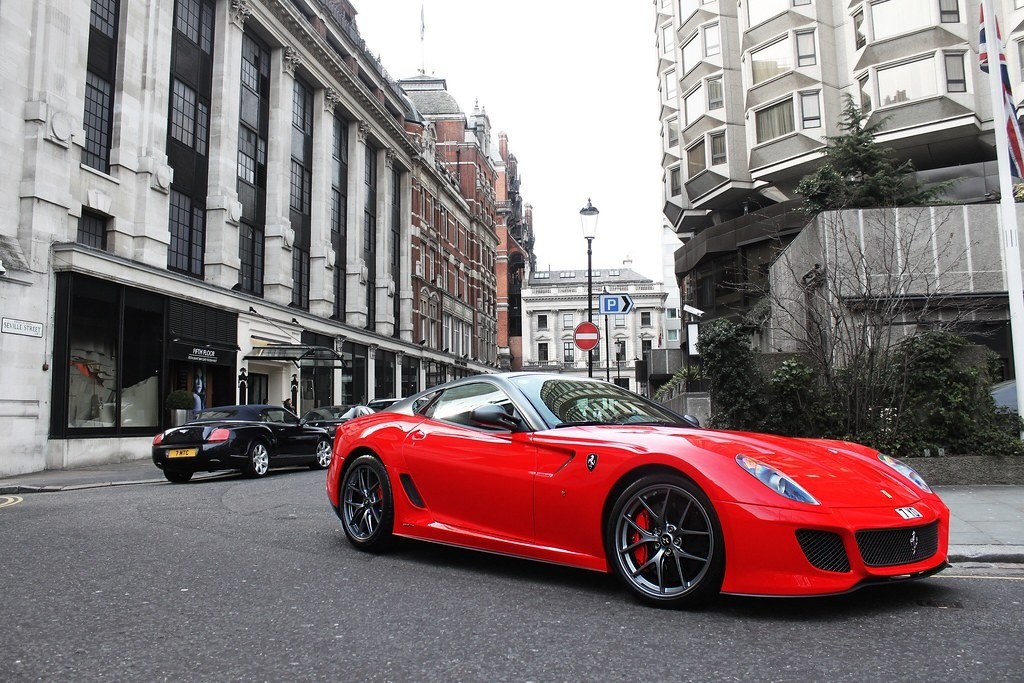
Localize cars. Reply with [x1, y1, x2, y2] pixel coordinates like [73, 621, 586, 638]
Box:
[297, 404, 376, 439]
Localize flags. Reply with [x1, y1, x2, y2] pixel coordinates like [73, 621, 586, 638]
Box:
[979, 2, 1024, 178]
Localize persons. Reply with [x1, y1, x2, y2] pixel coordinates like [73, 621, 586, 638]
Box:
[193, 365, 206, 410]
[283, 399, 295, 414]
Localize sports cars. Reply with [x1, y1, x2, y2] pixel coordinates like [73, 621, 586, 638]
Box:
[324, 371, 954, 610]
[149, 404, 331, 481]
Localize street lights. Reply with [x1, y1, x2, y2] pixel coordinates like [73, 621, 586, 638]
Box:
[614, 336, 624, 388]
[579, 197, 597, 377]
[601, 285, 614, 381]
[634, 355, 640, 395]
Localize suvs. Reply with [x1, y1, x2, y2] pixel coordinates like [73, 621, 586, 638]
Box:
[366, 396, 429, 416]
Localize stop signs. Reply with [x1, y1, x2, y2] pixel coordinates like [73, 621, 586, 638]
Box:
[573, 321, 602, 354]
